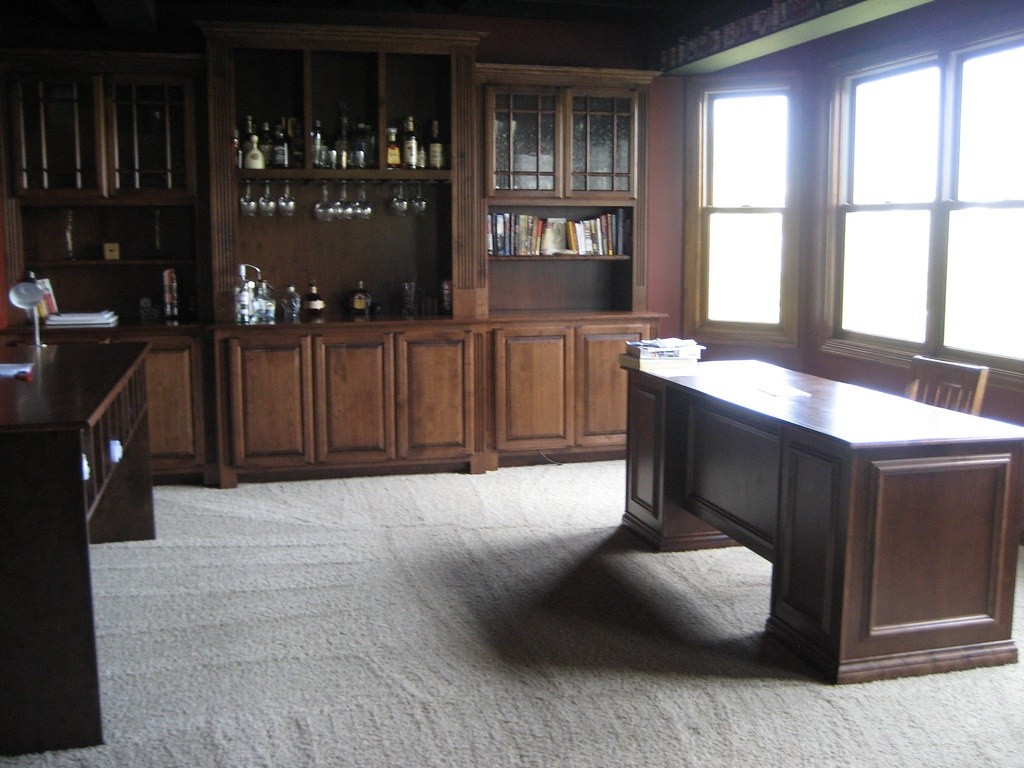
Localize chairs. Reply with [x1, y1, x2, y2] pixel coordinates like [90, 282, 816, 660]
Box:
[904, 355, 989, 416]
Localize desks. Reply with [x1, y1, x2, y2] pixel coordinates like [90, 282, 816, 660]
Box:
[0, 340, 155, 756]
[620, 359, 1024, 686]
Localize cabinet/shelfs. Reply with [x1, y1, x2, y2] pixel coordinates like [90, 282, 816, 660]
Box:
[0, 20, 663, 490]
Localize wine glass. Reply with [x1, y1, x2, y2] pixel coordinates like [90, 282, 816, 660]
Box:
[240, 177, 451, 222]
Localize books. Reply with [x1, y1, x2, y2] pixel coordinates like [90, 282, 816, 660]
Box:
[618, 338, 706, 370]
[488, 208, 631, 256]
[23, 279, 118, 328]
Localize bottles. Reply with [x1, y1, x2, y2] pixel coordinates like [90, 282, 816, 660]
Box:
[232, 110, 452, 170]
[234, 267, 453, 325]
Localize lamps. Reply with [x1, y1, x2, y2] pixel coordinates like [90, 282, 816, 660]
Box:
[9, 271, 47, 349]
